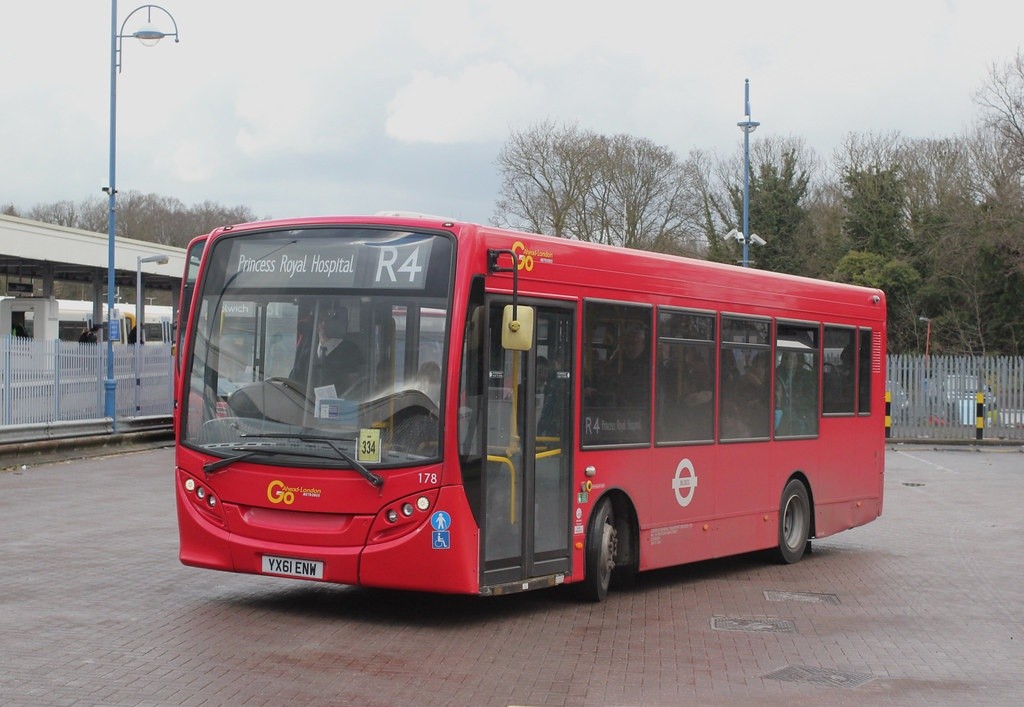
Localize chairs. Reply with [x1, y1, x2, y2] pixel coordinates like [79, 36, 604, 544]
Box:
[661, 358, 851, 437]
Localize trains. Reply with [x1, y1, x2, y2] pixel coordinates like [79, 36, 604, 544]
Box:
[23, 297, 173, 374]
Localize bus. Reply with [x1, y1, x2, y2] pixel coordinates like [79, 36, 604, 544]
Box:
[173, 219, 887, 605]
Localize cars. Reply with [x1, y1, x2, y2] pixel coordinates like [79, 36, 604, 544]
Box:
[888, 380, 909, 425]
[927, 374, 997, 413]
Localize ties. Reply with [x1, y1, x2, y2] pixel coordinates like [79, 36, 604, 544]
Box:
[319, 345, 328, 386]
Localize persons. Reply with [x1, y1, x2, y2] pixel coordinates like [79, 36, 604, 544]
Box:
[518, 321, 867, 441]
[79, 328, 98, 343]
[128, 322, 144, 344]
[286, 304, 362, 401]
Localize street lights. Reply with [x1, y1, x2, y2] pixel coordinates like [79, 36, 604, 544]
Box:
[104, 4, 179, 437]
[134, 255, 169, 414]
[919, 317, 932, 366]
[737, 79, 759, 269]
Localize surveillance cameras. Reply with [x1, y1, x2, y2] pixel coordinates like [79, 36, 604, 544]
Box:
[736, 232, 745, 243]
[102, 187, 110, 191]
[724, 229, 738, 240]
[750, 234, 767, 246]
